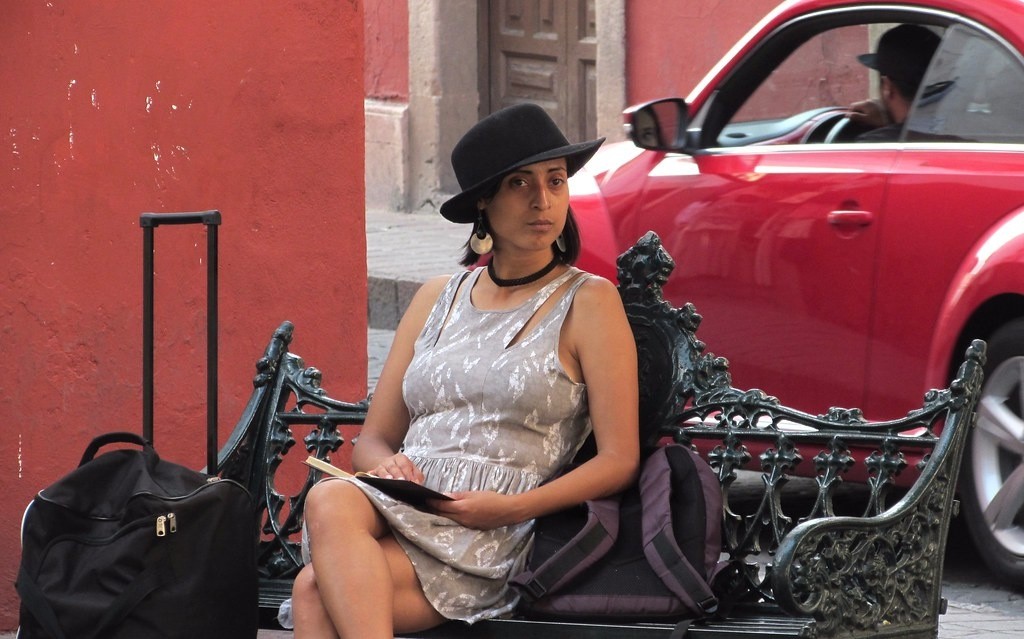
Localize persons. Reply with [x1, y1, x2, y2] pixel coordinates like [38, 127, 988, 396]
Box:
[632, 106, 665, 148]
[292, 103, 638, 639]
[845, 23, 942, 141]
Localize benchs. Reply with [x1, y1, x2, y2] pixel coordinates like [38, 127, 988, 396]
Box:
[201, 228, 989, 639]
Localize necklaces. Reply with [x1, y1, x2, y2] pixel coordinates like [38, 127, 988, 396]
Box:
[487, 241, 561, 287]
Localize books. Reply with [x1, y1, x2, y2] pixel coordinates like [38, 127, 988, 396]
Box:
[300, 456, 456, 501]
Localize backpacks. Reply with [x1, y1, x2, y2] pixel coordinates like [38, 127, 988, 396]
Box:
[507, 443, 723, 621]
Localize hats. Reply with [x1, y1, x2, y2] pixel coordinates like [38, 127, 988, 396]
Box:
[439, 103, 606, 223]
[857, 24, 943, 97]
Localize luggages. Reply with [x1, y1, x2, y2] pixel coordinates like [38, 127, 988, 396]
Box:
[14, 207, 262, 639]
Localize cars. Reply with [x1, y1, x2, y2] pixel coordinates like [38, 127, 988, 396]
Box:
[460, 0, 1024, 599]
